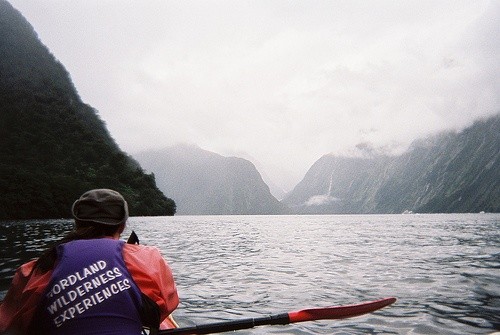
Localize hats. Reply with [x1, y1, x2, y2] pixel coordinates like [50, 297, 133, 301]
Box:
[71, 189, 128, 226]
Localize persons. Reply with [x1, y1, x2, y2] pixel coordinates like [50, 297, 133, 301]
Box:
[0, 187, 178, 335]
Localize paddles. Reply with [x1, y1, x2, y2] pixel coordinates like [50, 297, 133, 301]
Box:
[158, 298, 397, 335]
